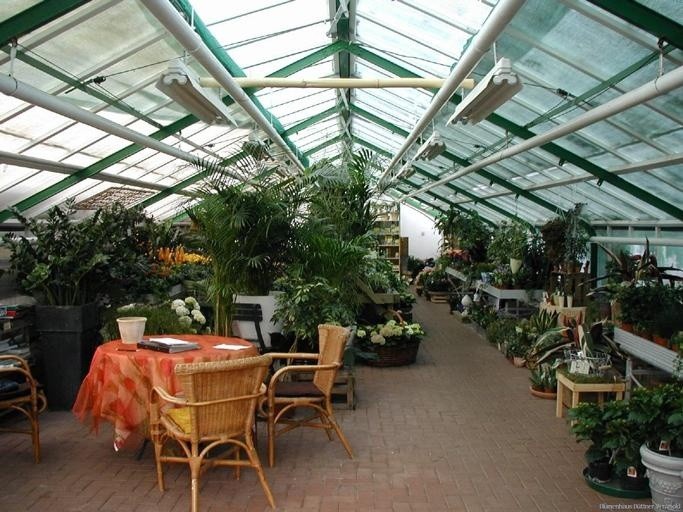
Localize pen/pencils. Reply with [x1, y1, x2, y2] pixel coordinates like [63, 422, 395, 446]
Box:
[116, 345, 139, 352]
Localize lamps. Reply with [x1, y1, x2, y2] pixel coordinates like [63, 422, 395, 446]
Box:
[385, 56, 523, 197]
[156, 59, 290, 178]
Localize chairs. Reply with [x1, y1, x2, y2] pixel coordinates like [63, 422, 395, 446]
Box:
[0, 350, 48, 462]
[152, 354, 276, 509]
[256, 325, 355, 468]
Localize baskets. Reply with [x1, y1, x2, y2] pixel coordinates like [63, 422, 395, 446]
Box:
[350, 309, 421, 365]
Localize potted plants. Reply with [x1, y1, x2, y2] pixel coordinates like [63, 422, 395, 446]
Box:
[427, 201, 683, 512]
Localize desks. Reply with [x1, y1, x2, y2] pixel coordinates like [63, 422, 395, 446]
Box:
[96, 335, 259, 461]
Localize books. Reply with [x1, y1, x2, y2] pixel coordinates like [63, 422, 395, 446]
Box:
[0, 305, 30, 366]
[136, 336, 199, 353]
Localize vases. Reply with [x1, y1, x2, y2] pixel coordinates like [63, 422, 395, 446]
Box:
[367, 338, 424, 369]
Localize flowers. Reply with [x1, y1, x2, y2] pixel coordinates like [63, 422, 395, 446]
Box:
[358, 312, 425, 343]
